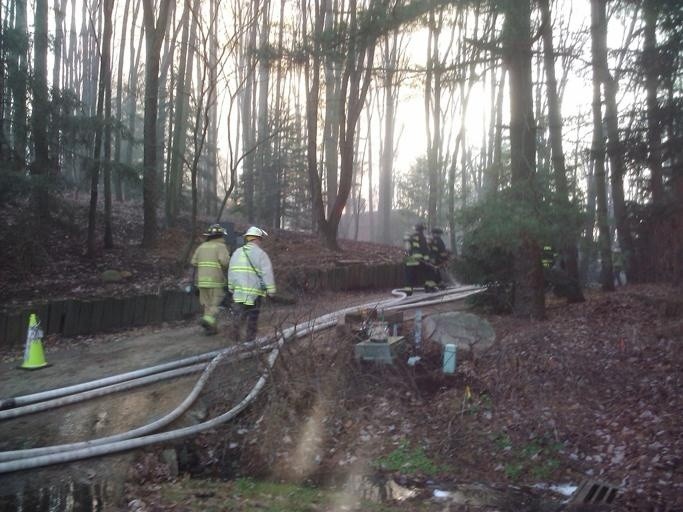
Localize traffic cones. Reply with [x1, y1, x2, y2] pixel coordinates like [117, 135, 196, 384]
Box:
[18, 313, 51, 371]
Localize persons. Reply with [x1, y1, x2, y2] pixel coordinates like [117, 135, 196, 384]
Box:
[403, 222, 439, 296]
[426, 227, 450, 290]
[191, 223, 230, 335]
[228, 226, 276, 344]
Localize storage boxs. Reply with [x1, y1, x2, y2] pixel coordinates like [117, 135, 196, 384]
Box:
[356, 336, 407, 365]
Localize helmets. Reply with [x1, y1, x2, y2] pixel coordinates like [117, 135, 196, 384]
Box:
[242, 227, 262, 237]
[202, 224, 227, 236]
